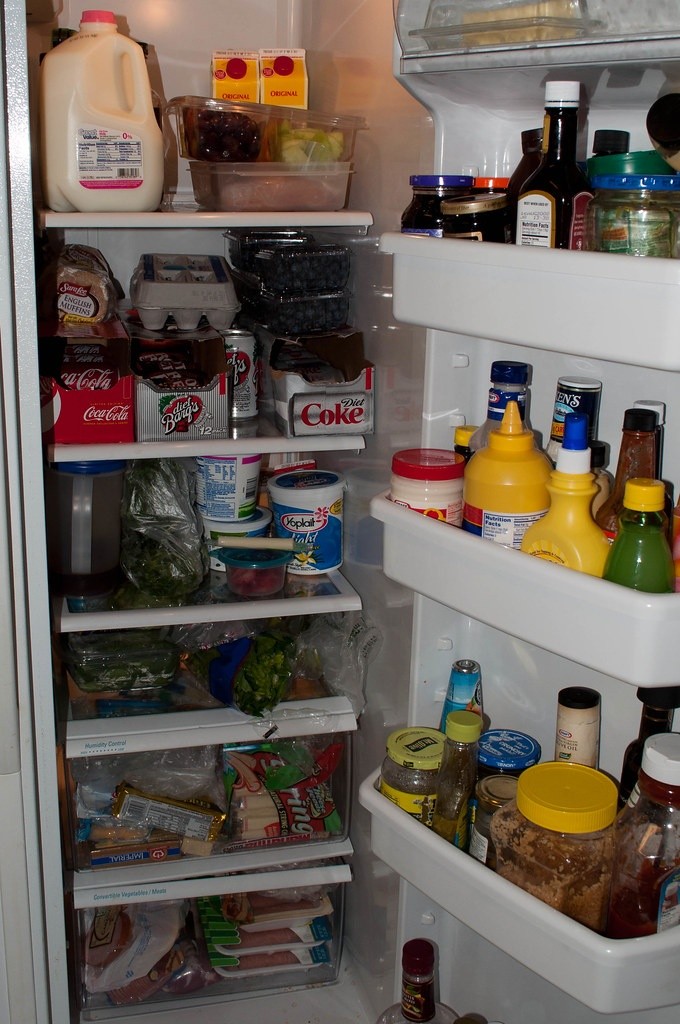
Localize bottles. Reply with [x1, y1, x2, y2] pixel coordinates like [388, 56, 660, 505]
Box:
[506, 126, 546, 243]
[375, 939, 460, 1024]
[38, 10, 164, 212]
[453, 356, 679, 593]
[431, 660, 680, 940]
[510, 81, 599, 251]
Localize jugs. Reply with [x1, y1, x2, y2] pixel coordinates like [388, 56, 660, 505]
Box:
[47, 460, 125, 599]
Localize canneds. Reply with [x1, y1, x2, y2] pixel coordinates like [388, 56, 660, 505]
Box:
[397, 168, 510, 242]
[387, 448, 465, 528]
[583, 168, 678, 258]
[380, 726, 444, 829]
[477, 729, 543, 776]
[221, 327, 261, 423]
